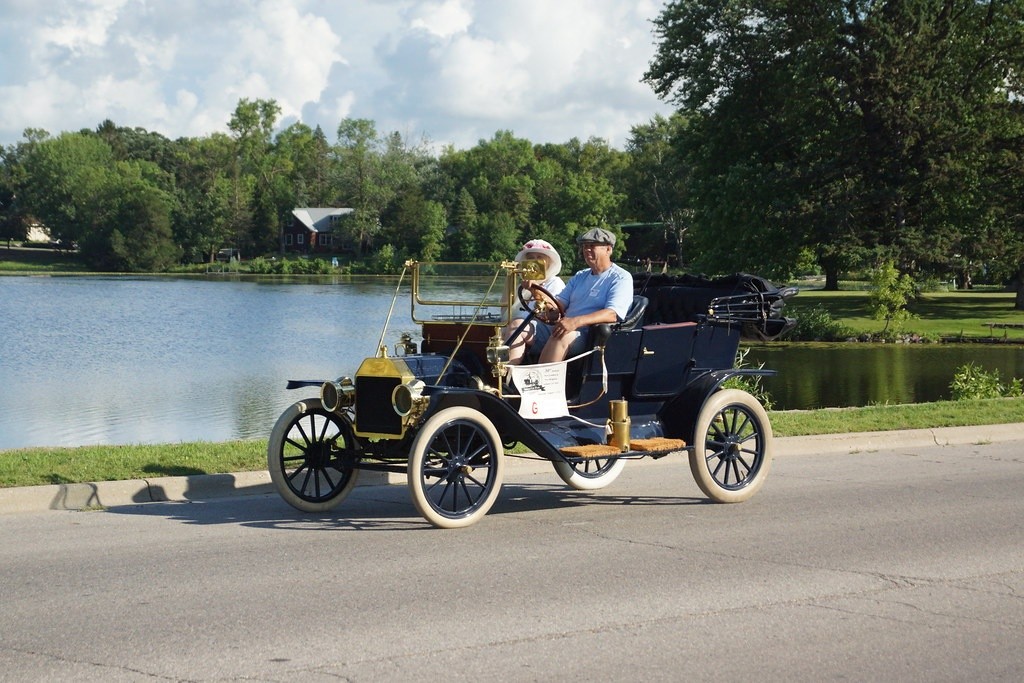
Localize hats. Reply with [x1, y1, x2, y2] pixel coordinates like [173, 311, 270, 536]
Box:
[515, 239, 561, 276]
[576, 228, 617, 248]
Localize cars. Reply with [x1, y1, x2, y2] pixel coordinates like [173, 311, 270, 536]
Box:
[268, 258, 800, 529]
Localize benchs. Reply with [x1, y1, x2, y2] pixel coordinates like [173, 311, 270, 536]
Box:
[582, 291, 650, 375]
[633, 285, 748, 331]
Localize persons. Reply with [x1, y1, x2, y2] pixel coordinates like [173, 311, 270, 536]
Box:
[505, 228, 634, 365]
[501, 239, 566, 340]
